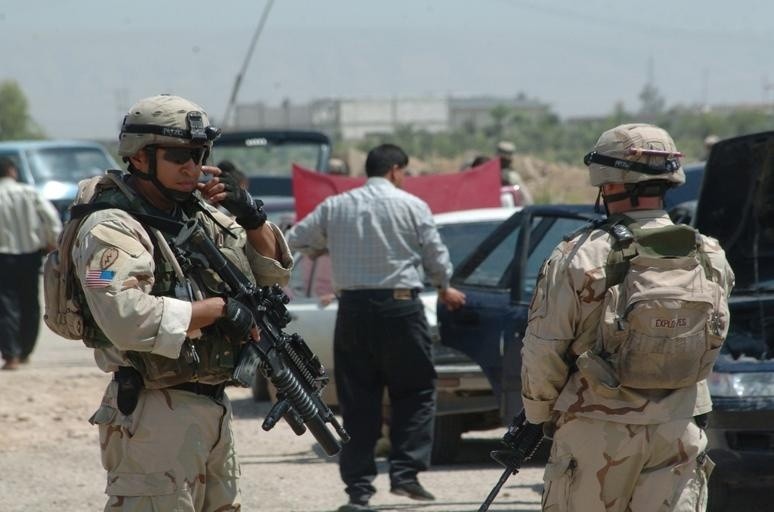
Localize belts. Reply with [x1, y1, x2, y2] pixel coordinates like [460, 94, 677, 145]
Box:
[111, 370, 226, 401]
[338, 287, 421, 302]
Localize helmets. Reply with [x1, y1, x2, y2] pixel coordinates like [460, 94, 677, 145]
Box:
[589, 122, 686, 187]
[116, 94, 212, 158]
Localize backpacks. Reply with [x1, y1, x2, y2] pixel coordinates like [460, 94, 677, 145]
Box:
[583, 216, 731, 391]
[43, 175, 111, 341]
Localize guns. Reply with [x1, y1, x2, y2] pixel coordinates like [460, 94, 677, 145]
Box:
[173, 218, 352, 456]
[478, 407, 544, 511]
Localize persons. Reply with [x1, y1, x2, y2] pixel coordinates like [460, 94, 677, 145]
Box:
[0, 157, 64, 373]
[497, 140, 535, 209]
[70, 88, 295, 512]
[212, 158, 251, 219]
[516, 120, 737, 512]
[286, 144, 470, 511]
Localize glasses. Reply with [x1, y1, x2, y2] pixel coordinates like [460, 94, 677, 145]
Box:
[137, 143, 204, 166]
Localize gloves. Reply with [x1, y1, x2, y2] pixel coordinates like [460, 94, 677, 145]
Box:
[515, 420, 545, 458]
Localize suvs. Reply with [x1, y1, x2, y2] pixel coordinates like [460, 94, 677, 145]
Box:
[204, 129, 359, 233]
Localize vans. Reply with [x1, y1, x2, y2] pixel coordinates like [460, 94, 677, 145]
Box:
[1, 139, 124, 228]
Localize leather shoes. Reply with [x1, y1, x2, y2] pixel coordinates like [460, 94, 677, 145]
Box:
[389, 476, 436, 501]
[346, 489, 373, 509]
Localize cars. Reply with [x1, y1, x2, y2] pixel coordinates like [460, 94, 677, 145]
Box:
[436, 131, 773, 511]
[252, 207, 543, 463]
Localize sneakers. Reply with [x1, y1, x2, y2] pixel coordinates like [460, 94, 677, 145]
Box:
[1, 354, 31, 370]
